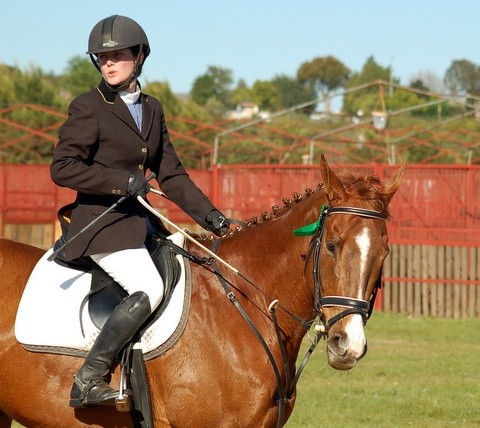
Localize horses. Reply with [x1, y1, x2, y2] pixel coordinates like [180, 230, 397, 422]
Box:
[1, 152, 407, 427]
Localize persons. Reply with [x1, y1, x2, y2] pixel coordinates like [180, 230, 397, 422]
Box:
[50, 15, 229, 407]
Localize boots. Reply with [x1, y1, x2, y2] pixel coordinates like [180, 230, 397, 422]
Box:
[69, 292, 152, 408]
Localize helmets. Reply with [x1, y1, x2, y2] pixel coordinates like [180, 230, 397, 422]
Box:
[86, 14, 150, 59]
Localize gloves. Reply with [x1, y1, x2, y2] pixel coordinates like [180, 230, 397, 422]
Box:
[206, 210, 230, 236]
[127, 173, 150, 199]
